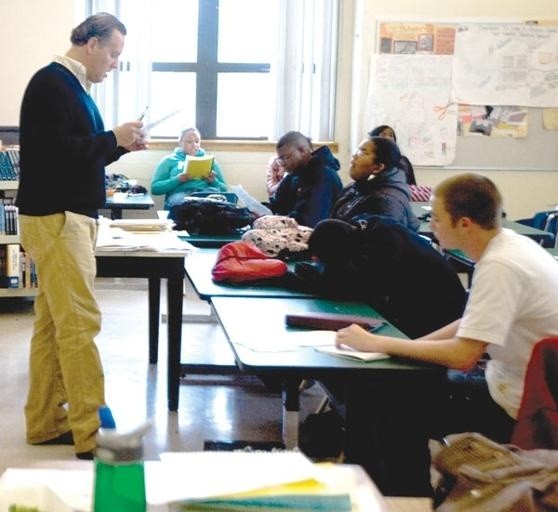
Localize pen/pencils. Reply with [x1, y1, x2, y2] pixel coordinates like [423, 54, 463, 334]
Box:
[139, 105, 150, 121]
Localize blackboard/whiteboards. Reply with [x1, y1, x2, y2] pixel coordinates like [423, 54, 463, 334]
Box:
[366, 17, 558, 171]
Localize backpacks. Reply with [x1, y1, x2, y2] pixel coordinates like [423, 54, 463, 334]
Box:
[168, 198, 257, 229]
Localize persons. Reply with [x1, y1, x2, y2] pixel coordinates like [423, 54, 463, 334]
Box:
[334, 169, 557, 496]
[13, 7, 151, 463]
[151, 125, 228, 219]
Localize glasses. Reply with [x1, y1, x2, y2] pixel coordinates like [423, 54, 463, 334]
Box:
[275, 148, 297, 166]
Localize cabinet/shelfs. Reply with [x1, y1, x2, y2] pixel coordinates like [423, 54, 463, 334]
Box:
[0, 179, 39, 299]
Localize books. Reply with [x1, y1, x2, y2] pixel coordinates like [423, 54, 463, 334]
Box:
[1, 139, 37, 288]
[313, 341, 391, 363]
[94, 212, 200, 256]
[184, 154, 216, 180]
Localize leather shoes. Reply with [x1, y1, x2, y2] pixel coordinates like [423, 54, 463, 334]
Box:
[32, 431, 93, 460]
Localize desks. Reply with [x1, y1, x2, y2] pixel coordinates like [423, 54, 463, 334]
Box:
[25, 193, 447, 511]
